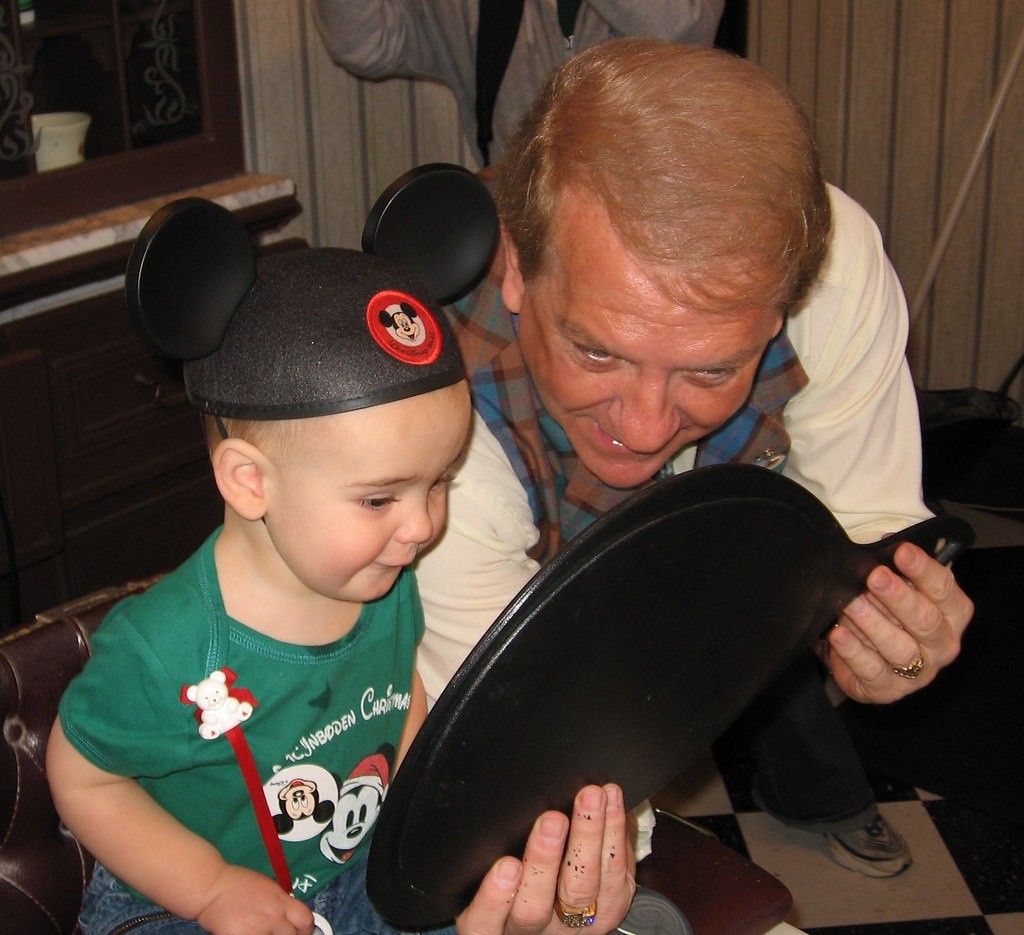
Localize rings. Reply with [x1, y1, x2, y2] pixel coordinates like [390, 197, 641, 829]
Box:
[892, 645, 925, 679]
[554, 896, 597, 928]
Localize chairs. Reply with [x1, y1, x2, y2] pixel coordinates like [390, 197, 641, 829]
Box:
[1, 571, 169, 933]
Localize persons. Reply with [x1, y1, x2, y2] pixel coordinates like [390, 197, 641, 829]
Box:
[46, 161, 501, 935]
[308, 0, 727, 170]
[415, 36, 975, 935]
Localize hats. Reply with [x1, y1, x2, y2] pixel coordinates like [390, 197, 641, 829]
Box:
[124, 163, 499, 420]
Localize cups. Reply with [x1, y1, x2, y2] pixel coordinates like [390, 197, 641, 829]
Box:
[32, 112, 91, 174]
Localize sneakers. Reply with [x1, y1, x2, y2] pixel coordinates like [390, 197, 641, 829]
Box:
[763, 809, 912, 877]
